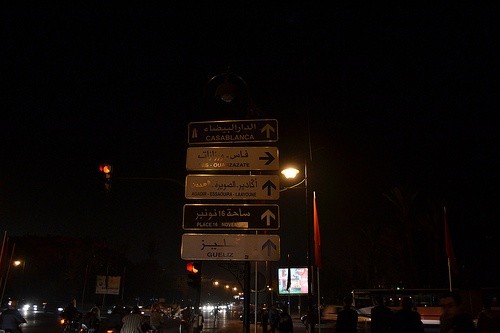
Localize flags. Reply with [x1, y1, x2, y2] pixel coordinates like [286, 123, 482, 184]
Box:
[312, 196, 321, 267]
[444, 210, 452, 262]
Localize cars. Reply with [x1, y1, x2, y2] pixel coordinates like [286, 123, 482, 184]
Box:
[4, 296, 244, 326]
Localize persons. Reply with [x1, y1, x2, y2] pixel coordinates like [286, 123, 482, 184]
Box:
[0, 271, 499, 333]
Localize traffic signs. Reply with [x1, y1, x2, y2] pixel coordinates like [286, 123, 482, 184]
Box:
[185, 174, 280, 200]
[187, 118, 280, 143]
[180, 233, 281, 261]
[185, 146, 280, 172]
[182, 203, 280, 230]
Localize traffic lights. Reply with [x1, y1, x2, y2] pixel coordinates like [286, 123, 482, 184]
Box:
[182, 262, 202, 289]
[97, 161, 115, 194]
